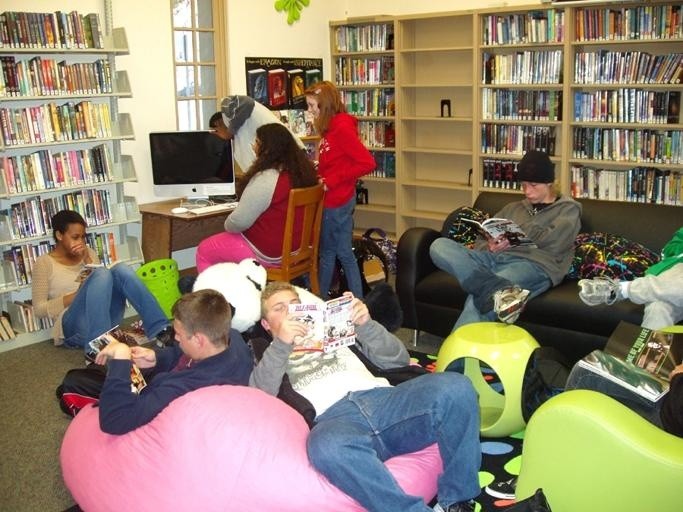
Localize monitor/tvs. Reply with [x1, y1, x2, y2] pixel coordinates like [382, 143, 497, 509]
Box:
[148, 130, 236, 202]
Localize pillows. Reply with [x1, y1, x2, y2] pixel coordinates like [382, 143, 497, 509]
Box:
[447, 205, 492, 252]
[564, 227, 661, 285]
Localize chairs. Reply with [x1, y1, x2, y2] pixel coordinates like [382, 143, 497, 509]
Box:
[260, 184, 325, 295]
[512, 326, 682, 512]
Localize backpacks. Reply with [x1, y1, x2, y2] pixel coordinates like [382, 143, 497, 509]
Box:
[337, 239, 389, 297]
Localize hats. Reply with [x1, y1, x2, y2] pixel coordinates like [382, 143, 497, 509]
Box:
[517, 150, 555, 183]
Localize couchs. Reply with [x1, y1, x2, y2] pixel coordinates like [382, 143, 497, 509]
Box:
[56, 384, 448, 512]
[394, 190, 682, 372]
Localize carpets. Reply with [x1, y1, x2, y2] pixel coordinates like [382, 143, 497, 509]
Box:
[402, 345, 524, 511]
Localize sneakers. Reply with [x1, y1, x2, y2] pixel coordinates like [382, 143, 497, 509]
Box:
[578, 276, 625, 307]
[484, 477, 516, 500]
[179, 274, 196, 293]
[155, 327, 178, 347]
[493, 285, 531, 323]
[433, 499, 476, 512]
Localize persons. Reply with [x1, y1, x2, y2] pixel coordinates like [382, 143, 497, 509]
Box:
[247, 280, 481, 511]
[176, 123, 318, 274]
[577, 228, 682, 331]
[32, 210, 172, 364]
[304, 81, 376, 299]
[428, 151, 582, 371]
[62, 289, 254, 436]
[484, 360, 682, 499]
[209, 95, 307, 174]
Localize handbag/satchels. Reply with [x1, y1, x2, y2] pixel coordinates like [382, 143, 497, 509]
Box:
[359, 227, 397, 271]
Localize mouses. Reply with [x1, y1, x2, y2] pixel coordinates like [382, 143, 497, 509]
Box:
[170, 207, 188, 214]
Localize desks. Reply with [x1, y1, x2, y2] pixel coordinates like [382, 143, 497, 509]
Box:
[138, 197, 239, 282]
[434, 320, 542, 438]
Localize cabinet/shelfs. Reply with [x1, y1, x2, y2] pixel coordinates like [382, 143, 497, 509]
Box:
[328, 0, 683, 257]
[0, 26, 147, 355]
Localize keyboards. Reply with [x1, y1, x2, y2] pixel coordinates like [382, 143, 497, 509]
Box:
[189, 202, 237, 215]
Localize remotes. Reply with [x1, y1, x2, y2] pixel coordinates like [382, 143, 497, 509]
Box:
[179, 203, 208, 207]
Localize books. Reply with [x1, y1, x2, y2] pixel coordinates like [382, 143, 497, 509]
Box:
[121, 320, 156, 345]
[479, 7, 564, 190]
[269, 111, 316, 161]
[570, 5, 683, 207]
[334, 25, 394, 204]
[288, 295, 356, 353]
[89, 324, 149, 395]
[461, 217, 537, 251]
[304, 68, 320, 89]
[73, 258, 129, 283]
[268, 67, 287, 108]
[286, 68, 304, 107]
[578, 320, 682, 402]
[248, 68, 267, 105]
[0, 11, 113, 342]
